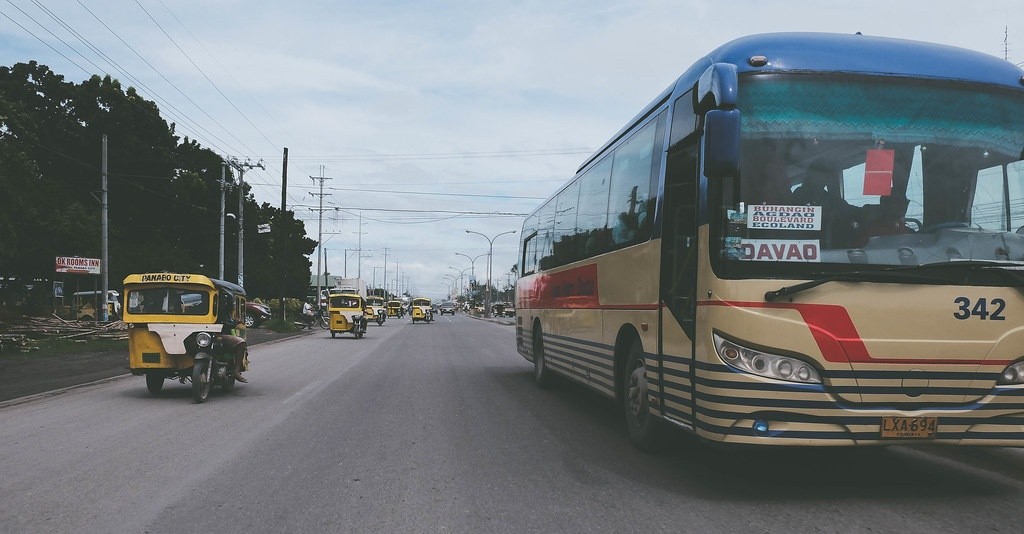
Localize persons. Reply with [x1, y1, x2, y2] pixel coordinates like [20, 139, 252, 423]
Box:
[793, 158, 916, 251]
[142, 290, 249, 383]
[303, 298, 318, 329]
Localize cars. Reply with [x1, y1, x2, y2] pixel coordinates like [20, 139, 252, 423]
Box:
[295, 288, 515, 330]
[246, 302, 272, 329]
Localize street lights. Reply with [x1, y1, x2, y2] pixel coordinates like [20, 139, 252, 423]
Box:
[441, 253, 491, 302]
[466, 230, 516, 318]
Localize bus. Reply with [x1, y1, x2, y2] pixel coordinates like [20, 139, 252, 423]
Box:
[512, 32, 1024, 455]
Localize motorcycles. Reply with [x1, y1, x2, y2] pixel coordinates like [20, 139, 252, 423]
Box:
[412, 298, 435, 324]
[123, 273, 250, 402]
[71, 290, 122, 321]
[328, 294, 368, 340]
